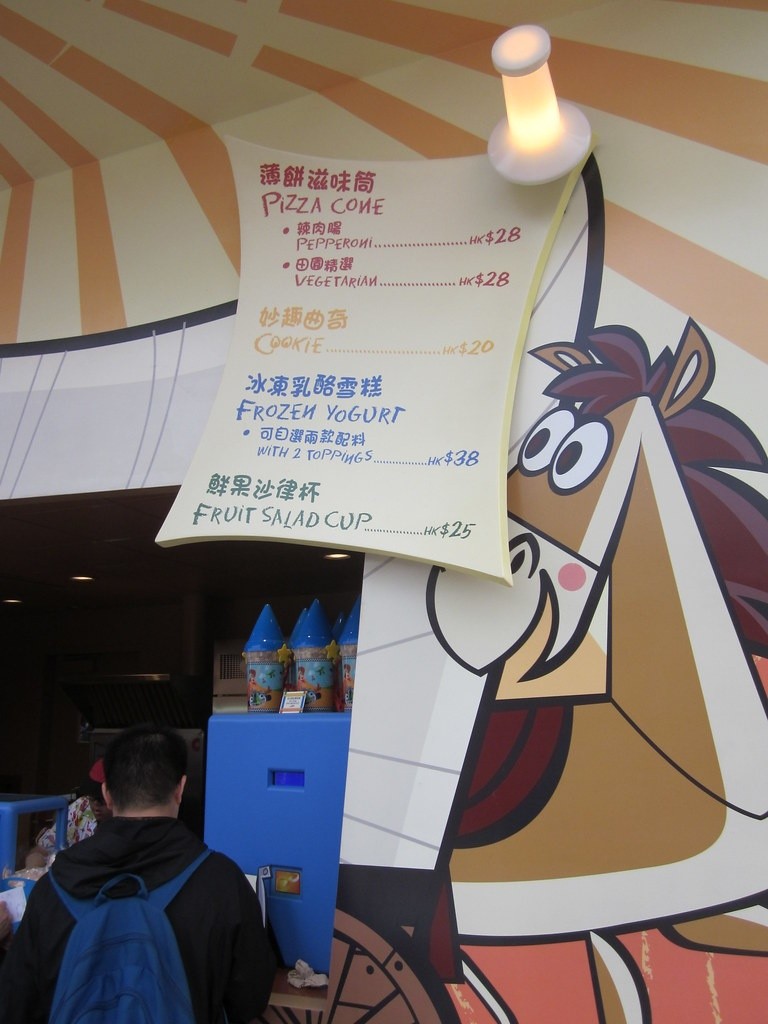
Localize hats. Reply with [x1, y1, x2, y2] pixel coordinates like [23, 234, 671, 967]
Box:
[88, 758, 106, 784]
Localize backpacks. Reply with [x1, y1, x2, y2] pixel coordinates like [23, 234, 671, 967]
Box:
[48, 849, 214, 1024]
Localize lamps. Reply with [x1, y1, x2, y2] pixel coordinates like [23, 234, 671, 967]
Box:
[488, 26, 591, 188]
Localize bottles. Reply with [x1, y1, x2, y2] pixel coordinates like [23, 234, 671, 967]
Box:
[241, 595, 361, 713]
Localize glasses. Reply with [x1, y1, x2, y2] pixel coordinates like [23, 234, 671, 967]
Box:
[86, 792, 106, 806]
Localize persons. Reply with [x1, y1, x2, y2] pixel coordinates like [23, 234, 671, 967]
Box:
[0, 723, 285, 1024]
